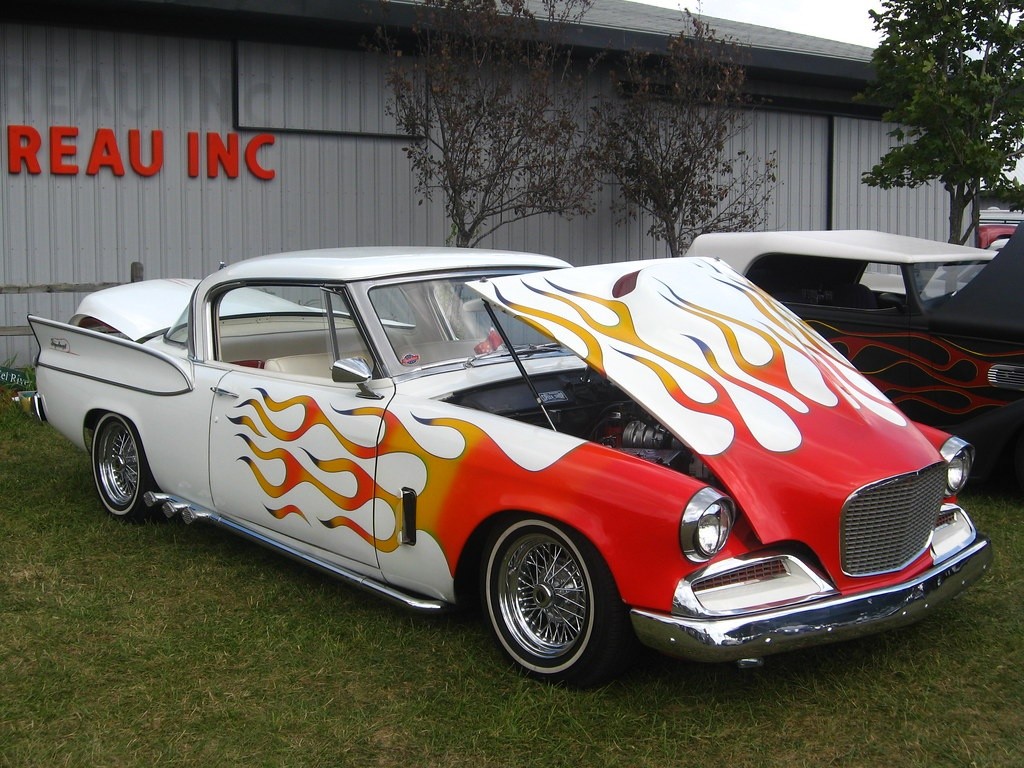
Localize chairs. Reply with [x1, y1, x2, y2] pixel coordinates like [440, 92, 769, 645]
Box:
[834, 283, 878, 309]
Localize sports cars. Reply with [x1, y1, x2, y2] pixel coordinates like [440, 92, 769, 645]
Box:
[26, 242, 993, 693]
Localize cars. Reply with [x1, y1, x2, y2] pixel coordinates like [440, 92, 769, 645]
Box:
[681, 209, 1024, 501]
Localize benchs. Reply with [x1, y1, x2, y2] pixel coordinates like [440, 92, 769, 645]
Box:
[263, 340, 504, 378]
